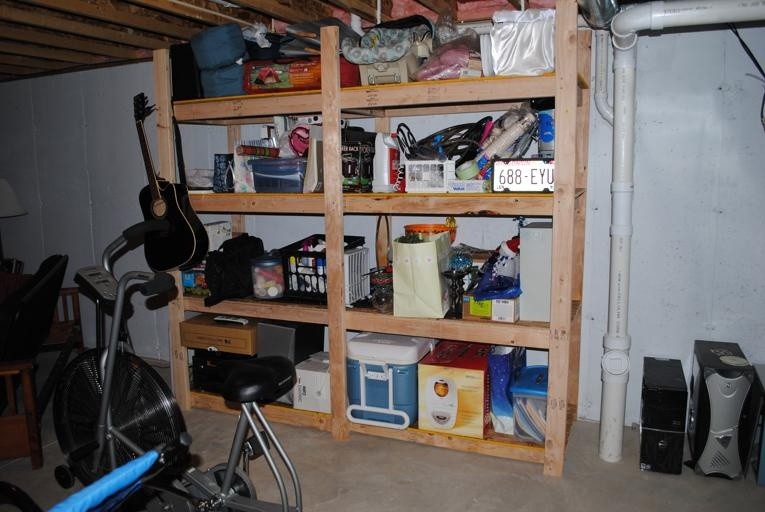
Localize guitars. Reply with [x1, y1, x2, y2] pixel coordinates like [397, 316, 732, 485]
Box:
[132, 91, 209, 273]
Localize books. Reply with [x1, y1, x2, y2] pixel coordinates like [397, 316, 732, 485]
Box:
[514, 396, 550, 439]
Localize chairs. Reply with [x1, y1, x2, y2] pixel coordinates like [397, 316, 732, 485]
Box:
[0, 270, 84, 471]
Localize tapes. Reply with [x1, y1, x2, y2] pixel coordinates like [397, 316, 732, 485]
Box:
[455, 160, 479, 179]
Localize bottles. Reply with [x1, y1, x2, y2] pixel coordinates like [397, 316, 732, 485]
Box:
[371, 130, 401, 194]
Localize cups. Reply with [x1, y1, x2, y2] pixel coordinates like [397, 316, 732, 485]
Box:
[470, 112, 536, 178]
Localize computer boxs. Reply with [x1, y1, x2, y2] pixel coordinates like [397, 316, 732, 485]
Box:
[640, 357, 688, 474]
[684, 340, 765, 480]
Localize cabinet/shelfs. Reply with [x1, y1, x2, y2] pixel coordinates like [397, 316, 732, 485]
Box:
[154, 0, 593, 477]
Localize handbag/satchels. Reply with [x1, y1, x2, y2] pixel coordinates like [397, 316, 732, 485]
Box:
[212, 154, 235, 193]
[489, 345, 526, 435]
[204, 232, 264, 307]
[391, 231, 451, 318]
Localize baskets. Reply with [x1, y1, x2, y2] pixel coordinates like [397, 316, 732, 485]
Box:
[404, 160, 455, 193]
[280, 234, 366, 302]
[404, 224, 458, 244]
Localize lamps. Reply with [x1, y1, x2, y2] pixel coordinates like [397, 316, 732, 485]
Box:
[0, 177, 28, 274]
